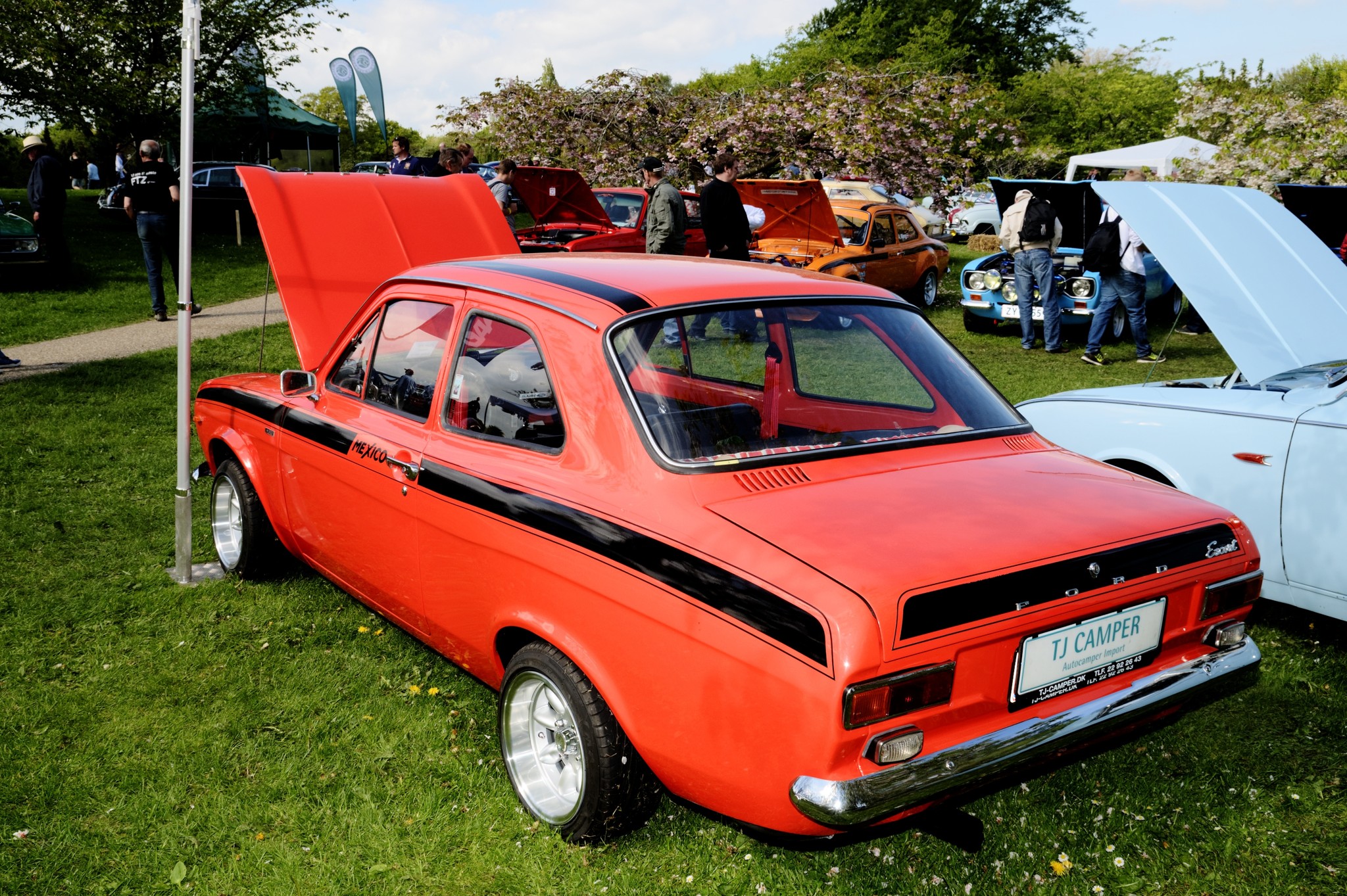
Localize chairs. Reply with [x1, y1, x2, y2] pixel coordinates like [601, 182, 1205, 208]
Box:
[855, 219, 887, 247]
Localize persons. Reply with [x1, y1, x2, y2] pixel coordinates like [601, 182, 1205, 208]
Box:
[122, 139, 201, 322]
[999, 189, 1071, 352]
[1174, 300, 1203, 336]
[686, 154, 768, 341]
[390, 137, 518, 234]
[1084, 168, 1101, 182]
[69, 142, 127, 190]
[637, 156, 689, 347]
[1080, 170, 1167, 366]
[21, 135, 66, 221]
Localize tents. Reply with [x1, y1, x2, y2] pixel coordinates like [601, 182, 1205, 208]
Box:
[1065, 136, 1220, 182]
[173, 82, 341, 170]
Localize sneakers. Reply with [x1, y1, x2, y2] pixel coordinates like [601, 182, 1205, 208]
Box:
[1081, 352, 1109, 366]
[1138, 351, 1166, 364]
[1174, 325, 1198, 337]
[0, 350, 22, 368]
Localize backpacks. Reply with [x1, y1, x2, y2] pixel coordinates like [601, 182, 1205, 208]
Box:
[1017, 197, 1056, 242]
[1083, 208, 1134, 272]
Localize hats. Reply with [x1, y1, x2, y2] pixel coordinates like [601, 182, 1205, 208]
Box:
[638, 156, 663, 172]
[20, 136, 48, 154]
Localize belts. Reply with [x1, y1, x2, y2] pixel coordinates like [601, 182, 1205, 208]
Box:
[140, 210, 157, 215]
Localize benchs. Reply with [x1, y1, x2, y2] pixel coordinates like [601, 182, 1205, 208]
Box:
[647, 402, 765, 464]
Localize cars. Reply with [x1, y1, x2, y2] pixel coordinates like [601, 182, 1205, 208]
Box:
[917, 180, 1002, 238]
[349, 162, 392, 174]
[193, 167, 1266, 846]
[819, 180, 956, 242]
[701, 180, 952, 306]
[984, 180, 1347, 622]
[500, 165, 709, 260]
[960, 176, 1184, 329]
[467, 163, 525, 207]
[96, 161, 279, 228]
[0, 200, 48, 271]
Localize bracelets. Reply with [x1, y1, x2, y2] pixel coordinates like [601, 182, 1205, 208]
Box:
[507, 208, 511, 214]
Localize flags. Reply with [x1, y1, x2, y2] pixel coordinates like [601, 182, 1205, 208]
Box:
[348, 47, 387, 143]
[329, 58, 357, 142]
[233, 41, 267, 119]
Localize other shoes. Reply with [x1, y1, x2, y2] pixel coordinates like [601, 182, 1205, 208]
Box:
[155, 311, 166, 321]
[1046, 346, 1070, 353]
[722, 330, 736, 335]
[688, 334, 709, 341]
[741, 335, 768, 343]
[1023, 346, 1030, 349]
[191, 304, 202, 315]
[650, 338, 682, 349]
